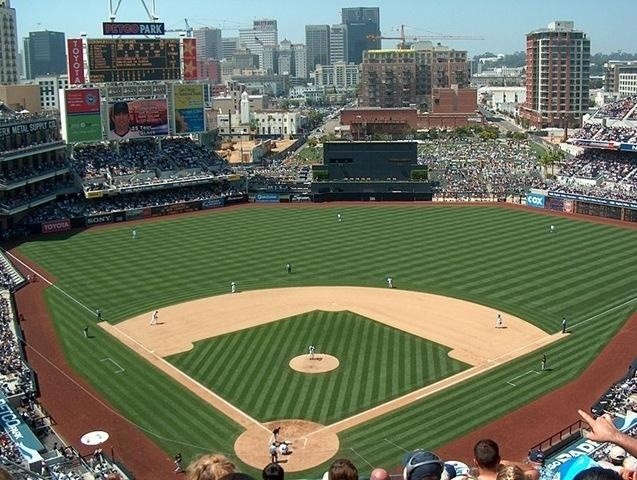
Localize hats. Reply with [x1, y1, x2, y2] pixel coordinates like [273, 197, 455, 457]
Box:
[404, 449, 441, 480]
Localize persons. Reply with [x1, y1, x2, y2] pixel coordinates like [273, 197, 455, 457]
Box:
[0, 94, 637, 241]
[492, 311, 507, 329]
[146, 308, 161, 327]
[307, 343, 316, 361]
[186, 451, 285, 480]
[401, 438, 541, 480]
[560, 351, 635, 476]
[267, 422, 284, 439]
[0, 249, 124, 476]
[269, 440, 289, 463]
[285, 259, 293, 272]
[538, 353, 551, 374]
[224, 279, 243, 298]
[322, 459, 359, 480]
[382, 273, 396, 290]
[370, 468, 391, 480]
[558, 317, 570, 333]
[173, 451, 183, 473]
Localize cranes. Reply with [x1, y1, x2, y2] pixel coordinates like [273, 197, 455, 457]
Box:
[163, 18, 255, 38]
[366, 24, 486, 49]
